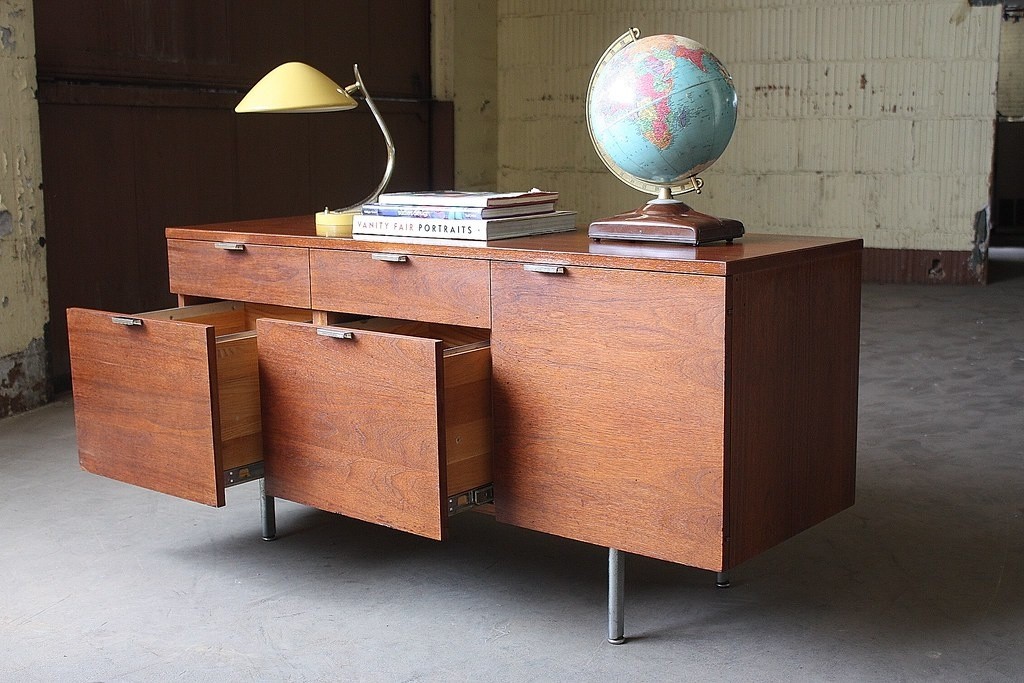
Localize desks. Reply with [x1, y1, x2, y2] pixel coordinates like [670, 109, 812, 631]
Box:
[65, 210, 865, 647]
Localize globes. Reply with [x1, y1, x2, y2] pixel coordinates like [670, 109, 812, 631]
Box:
[581, 24, 749, 247]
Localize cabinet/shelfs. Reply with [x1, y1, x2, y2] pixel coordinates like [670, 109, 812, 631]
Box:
[33, 0, 456, 376]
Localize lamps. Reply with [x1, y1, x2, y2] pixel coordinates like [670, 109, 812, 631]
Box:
[235, 61, 396, 237]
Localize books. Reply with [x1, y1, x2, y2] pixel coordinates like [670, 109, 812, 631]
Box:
[352, 188, 578, 240]
